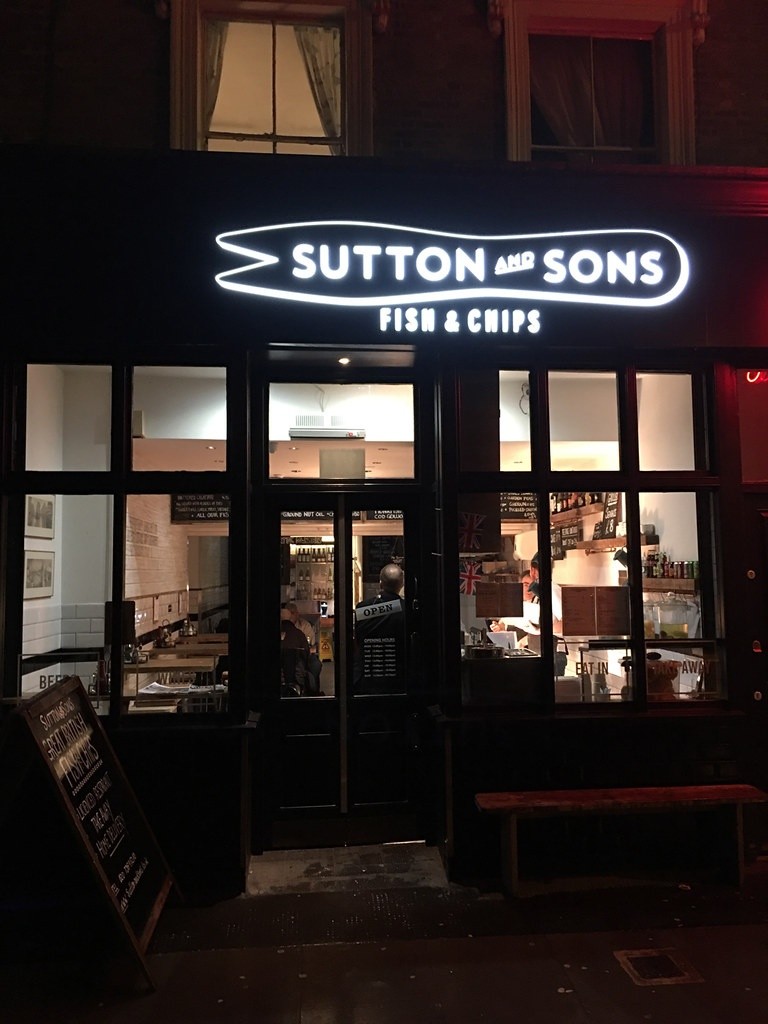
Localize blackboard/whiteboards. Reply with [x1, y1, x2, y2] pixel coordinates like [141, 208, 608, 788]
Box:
[25, 677, 177, 965]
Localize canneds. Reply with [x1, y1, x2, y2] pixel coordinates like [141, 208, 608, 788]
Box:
[653, 560, 698, 578]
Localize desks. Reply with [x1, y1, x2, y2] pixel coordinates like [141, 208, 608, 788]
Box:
[20, 632, 230, 714]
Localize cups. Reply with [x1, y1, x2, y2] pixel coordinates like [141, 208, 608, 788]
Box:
[593, 673, 606, 702]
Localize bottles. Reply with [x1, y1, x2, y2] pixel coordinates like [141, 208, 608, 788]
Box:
[321, 603, 328, 618]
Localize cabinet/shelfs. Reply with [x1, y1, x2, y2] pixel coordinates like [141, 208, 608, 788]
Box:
[616, 565, 701, 701]
[296, 541, 334, 625]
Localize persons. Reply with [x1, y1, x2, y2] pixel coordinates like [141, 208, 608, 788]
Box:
[281, 603, 323, 697]
[490, 552, 569, 676]
[354, 563, 405, 695]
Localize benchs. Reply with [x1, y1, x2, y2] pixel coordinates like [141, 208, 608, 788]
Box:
[474, 784, 768, 901]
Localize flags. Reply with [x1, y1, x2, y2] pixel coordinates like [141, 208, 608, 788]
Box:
[459, 560, 482, 596]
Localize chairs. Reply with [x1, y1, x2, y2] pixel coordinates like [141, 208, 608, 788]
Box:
[283, 644, 323, 696]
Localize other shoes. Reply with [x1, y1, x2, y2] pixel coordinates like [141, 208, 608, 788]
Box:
[320, 691, 325, 696]
